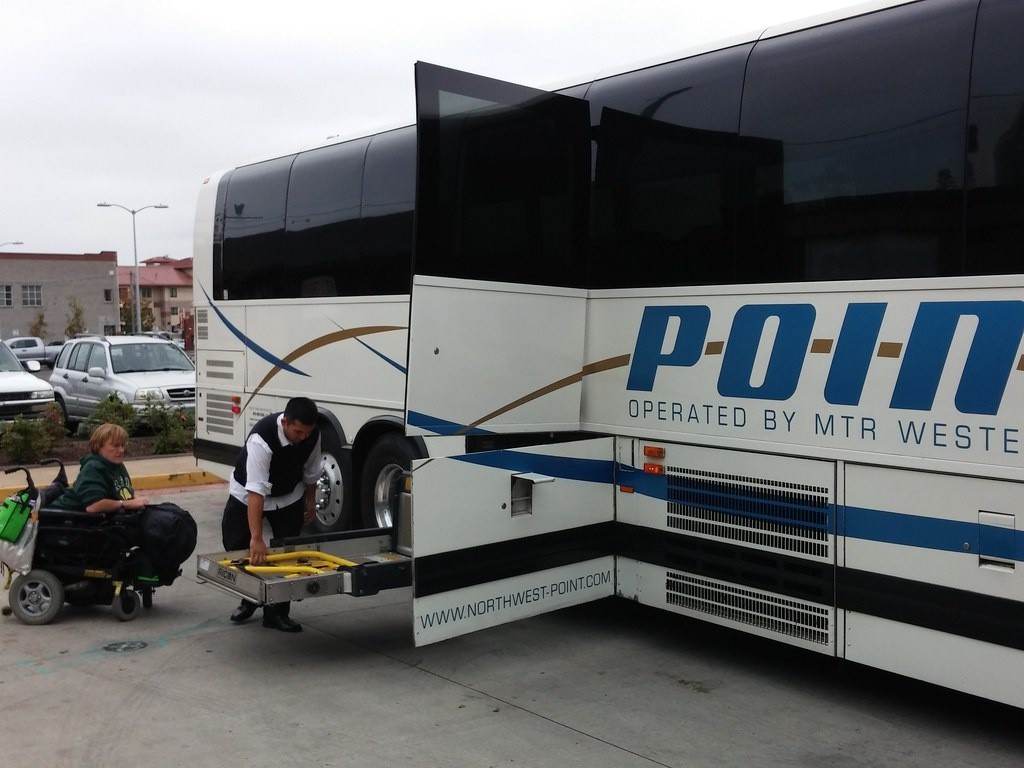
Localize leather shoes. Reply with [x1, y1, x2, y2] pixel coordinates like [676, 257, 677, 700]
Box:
[262, 615, 303, 632]
[229, 602, 256, 622]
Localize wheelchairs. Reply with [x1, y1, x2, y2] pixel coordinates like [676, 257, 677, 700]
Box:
[1, 456, 184, 625]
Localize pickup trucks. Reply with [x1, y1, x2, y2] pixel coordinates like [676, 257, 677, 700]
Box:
[6, 336, 66, 369]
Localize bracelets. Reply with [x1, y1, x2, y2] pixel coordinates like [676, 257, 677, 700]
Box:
[120, 499, 125, 508]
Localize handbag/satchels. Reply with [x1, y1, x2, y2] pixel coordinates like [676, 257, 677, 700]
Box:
[0, 489, 42, 576]
[0, 487, 33, 542]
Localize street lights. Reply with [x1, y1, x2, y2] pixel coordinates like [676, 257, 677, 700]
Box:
[97, 202, 169, 333]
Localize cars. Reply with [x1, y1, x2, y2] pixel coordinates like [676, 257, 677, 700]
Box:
[71, 330, 186, 350]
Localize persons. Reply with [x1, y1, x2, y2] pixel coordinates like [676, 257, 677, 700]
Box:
[51, 423, 197, 593]
[221, 397, 324, 631]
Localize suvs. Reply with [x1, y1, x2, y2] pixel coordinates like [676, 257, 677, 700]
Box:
[49, 332, 196, 437]
[0, 339, 55, 421]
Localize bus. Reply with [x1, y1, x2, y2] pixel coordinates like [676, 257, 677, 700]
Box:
[187, 2, 1024, 722]
